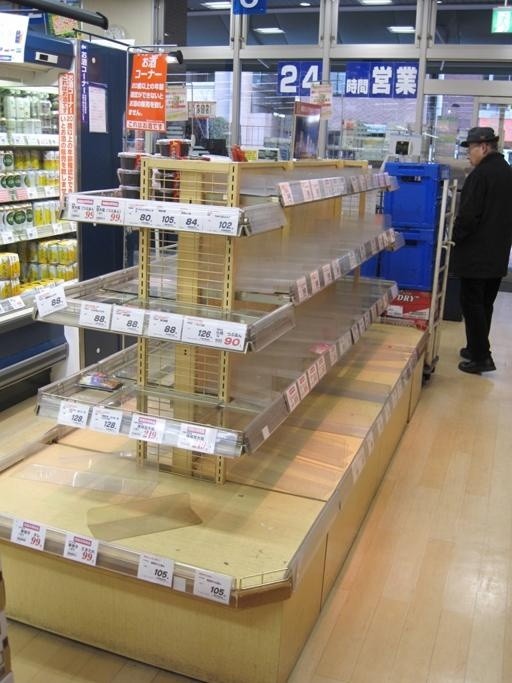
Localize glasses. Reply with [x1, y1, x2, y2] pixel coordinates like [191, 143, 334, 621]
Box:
[466, 144, 480, 155]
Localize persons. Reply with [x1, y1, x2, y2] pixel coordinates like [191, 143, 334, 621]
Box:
[449, 126, 512, 375]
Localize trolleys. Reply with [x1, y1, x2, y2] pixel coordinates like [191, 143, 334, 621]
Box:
[422, 173, 459, 388]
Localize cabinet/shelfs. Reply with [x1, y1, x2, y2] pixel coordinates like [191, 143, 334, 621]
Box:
[3, 154, 431, 683]
[0, 29, 77, 471]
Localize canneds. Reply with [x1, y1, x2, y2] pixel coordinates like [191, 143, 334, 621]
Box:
[0, 238, 80, 301]
[0, 149, 60, 188]
[0, 200, 60, 232]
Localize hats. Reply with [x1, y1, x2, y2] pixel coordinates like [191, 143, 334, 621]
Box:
[460, 126, 500, 147]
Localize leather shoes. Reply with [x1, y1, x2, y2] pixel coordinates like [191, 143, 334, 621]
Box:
[460, 347, 471, 360]
[458, 357, 497, 373]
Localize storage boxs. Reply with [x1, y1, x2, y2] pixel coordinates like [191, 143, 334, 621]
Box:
[384, 163, 449, 228]
[377, 288, 437, 320]
[360, 254, 379, 277]
[380, 229, 438, 290]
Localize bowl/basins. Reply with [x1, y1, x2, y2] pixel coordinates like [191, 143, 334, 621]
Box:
[118, 150, 149, 171]
[118, 186, 140, 199]
[117, 168, 142, 186]
[152, 168, 175, 202]
[156, 138, 192, 156]
